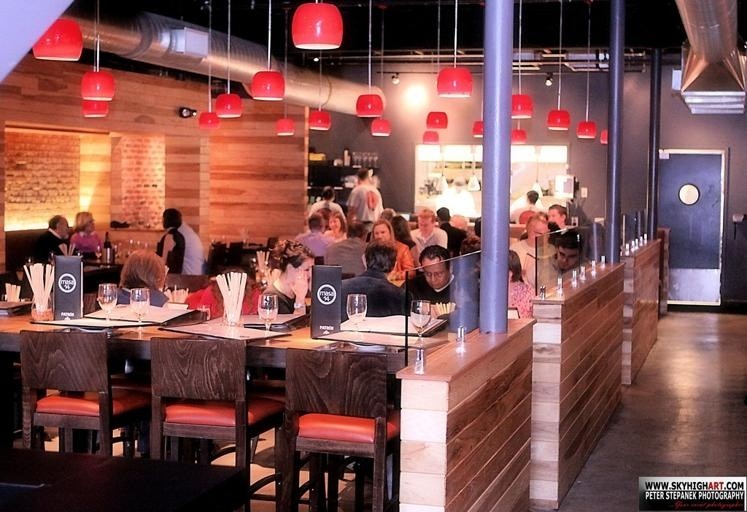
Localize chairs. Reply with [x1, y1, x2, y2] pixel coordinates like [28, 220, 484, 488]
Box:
[20, 331, 150, 457]
[150, 337, 284, 512]
[342, 274, 354, 279]
[275, 348, 399, 511]
[208, 236, 279, 273]
[315, 256, 324, 265]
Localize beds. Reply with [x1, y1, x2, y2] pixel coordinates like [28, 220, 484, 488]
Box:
[0, 317, 458, 462]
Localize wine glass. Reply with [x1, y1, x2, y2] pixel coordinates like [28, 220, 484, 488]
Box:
[26, 257, 34, 267]
[48, 252, 55, 266]
[346, 294, 367, 339]
[410, 300, 432, 346]
[97, 283, 117, 324]
[130, 288, 151, 326]
[257, 295, 279, 342]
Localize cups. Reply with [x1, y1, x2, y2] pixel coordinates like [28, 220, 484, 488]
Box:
[96, 244, 102, 263]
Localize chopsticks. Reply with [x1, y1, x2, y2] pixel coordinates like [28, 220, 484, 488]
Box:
[256, 250, 270, 274]
[4, 283, 20, 303]
[216, 272, 246, 324]
[21, 263, 55, 314]
[433, 301, 455, 317]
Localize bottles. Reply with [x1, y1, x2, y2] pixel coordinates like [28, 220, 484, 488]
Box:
[353, 152, 378, 167]
[103, 233, 112, 264]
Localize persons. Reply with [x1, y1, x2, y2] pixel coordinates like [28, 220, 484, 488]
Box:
[295, 212, 335, 257]
[257, 239, 315, 315]
[120, 249, 189, 308]
[519, 212, 549, 240]
[265, 236, 285, 273]
[409, 209, 449, 268]
[324, 221, 368, 275]
[461, 217, 481, 255]
[516, 191, 540, 225]
[437, 207, 468, 258]
[177, 223, 205, 275]
[390, 215, 420, 267]
[548, 222, 561, 233]
[340, 238, 413, 322]
[362, 220, 417, 280]
[508, 250, 535, 319]
[58, 211, 102, 256]
[33, 215, 102, 264]
[316, 208, 334, 243]
[323, 211, 347, 245]
[508, 215, 559, 296]
[400, 244, 455, 317]
[309, 186, 347, 223]
[347, 167, 384, 224]
[548, 204, 575, 231]
[379, 208, 396, 221]
[554, 229, 590, 275]
[155, 208, 185, 289]
[183, 265, 265, 320]
